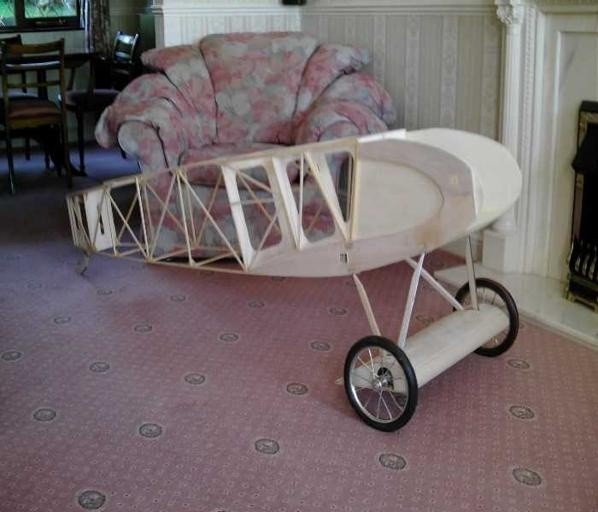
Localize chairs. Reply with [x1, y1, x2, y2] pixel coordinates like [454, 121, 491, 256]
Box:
[0, 34, 77, 191]
[56, 31, 141, 172]
[92, 29, 397, 262]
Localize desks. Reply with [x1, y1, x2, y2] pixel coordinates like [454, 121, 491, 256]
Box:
[0, 48, 104, 192]
